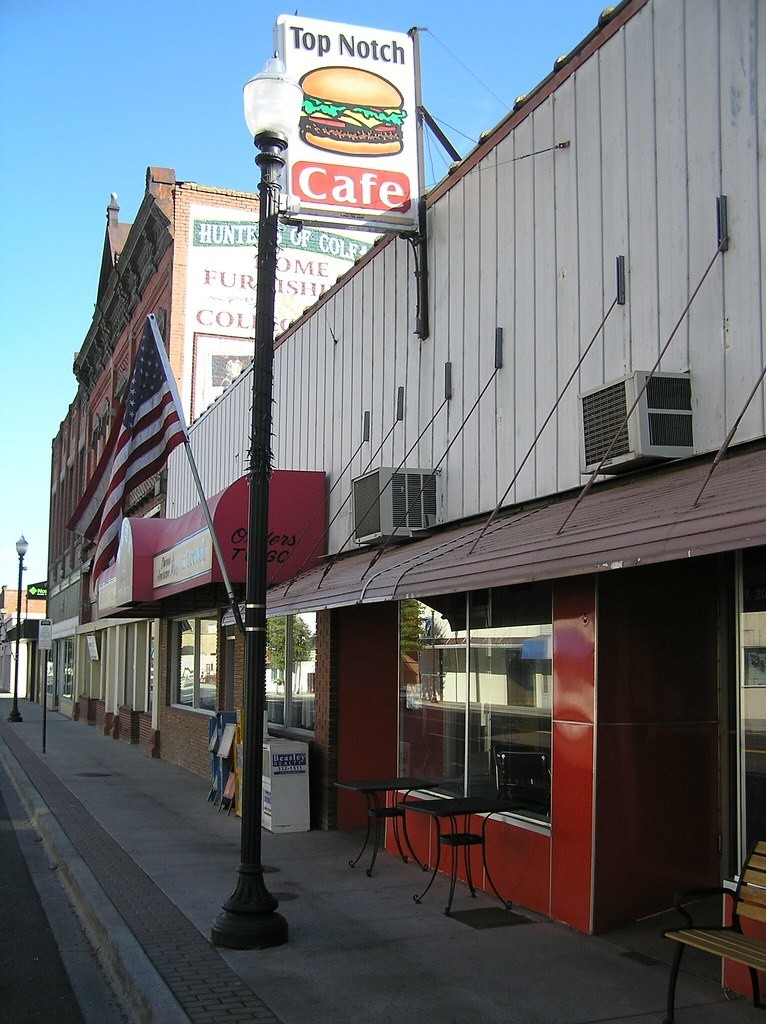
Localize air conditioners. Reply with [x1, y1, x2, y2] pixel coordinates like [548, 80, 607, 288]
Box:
[350, 467, 442, 545]
[577, 370, 693, 475]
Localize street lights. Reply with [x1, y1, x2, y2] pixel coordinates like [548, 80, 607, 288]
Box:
[206, 58, 305, 952]
[7, 535, 28, 723]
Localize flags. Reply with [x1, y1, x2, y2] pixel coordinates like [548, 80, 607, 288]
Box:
[66, 313, 189, 593]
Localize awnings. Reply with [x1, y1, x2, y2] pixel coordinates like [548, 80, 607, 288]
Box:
[222, 435, 766, 626]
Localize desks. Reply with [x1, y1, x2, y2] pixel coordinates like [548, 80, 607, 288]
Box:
[333, 777, 439, 878]
[396, 796, 527, 918]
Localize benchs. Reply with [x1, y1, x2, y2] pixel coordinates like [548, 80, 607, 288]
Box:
[658, 837, 766, 1024]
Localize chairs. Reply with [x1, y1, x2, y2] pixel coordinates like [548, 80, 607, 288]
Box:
[499, 751, 550, 818]
[493, 744, 551, 799]
